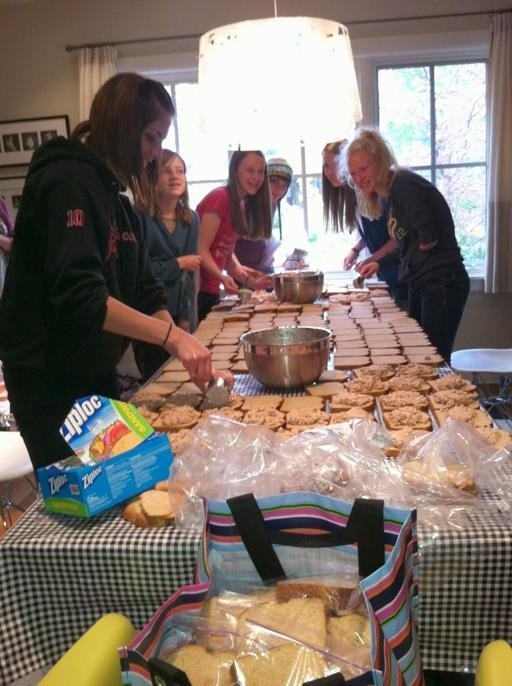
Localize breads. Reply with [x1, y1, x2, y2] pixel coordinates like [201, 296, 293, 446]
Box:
[128, 283, 512, 457]
[163, 576, 371, 686]
[123, 480, 176, 528]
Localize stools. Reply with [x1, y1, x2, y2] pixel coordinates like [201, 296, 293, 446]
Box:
[1, 430, 38, 531]
[450, 346, 512, 430]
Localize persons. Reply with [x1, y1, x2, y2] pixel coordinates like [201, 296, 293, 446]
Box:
[0, 73, 235, 483]
[0, 197, 15, 299]
[132, 148, 200, 382]
[334, 128, 470, 368]
[321, 139, 408, 314]
[195, 151, 273, 324]
[234, 158, 293, 293]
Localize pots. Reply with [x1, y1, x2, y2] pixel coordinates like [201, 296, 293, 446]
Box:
[240, 325, 336, 389]
[271, 271, 324, 305]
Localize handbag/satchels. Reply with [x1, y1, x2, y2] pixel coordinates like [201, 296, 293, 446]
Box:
[117, 489, 428, 686]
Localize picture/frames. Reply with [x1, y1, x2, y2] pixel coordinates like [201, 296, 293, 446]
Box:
[1, 114, 72, 237]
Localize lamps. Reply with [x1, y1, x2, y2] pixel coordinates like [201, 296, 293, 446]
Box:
[197, 0, 363, 151]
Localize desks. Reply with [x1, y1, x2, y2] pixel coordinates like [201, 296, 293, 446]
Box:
[1, 269, 512, 686]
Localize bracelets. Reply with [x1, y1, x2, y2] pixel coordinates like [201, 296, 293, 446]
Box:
[162, 323, 173, 348]
[245, 276, 251, 287]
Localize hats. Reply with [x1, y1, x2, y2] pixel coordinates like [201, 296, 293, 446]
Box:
[267, 157, 293, 181]
